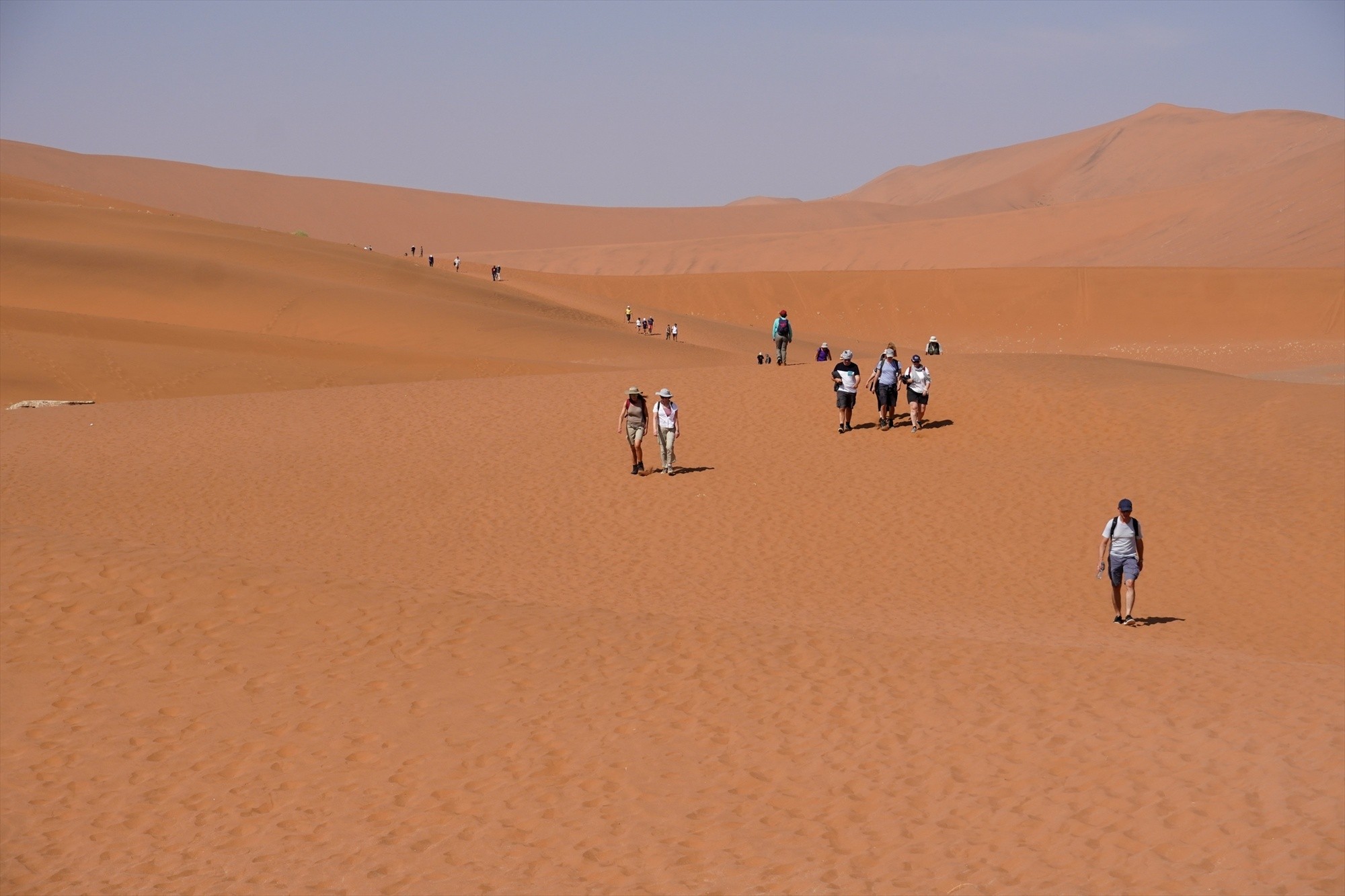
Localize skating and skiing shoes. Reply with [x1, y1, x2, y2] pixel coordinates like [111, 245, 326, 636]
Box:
[777, 317, 789, 336]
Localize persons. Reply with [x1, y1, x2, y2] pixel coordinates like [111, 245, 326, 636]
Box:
[618, 387, 648, 475]
[1097, 498, 1144, 625]
[772, 310, 792, 366]
[653, 388, 680, 475]
[492, 265, 501, 281]
[665, 323, 677, 341]
[411, 246, 415, 257]
[420, 246, 423, 257]
[429, 255, 434, 267]
[636, 316, 653, 335]
[364, 245, 372, 251]
[831, 350, 861, 433]
[866, 342, 902, 428]
[757, 352, 771, 364]
[625, 306, 632, 323]
[925, 336, 943, 355]
[454, 256, 460, 272]
[905, 355, 931, 433]
[816, 342, 832, 362]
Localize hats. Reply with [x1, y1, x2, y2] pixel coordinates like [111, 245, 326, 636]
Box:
[886, 348, 894, 357]
[779, 310, 787, 316]
[625, 387, 643, 395]
[1118, 498, 1133, 512]
[882, 349, 886, 358]
[821, 343, 829, 348]
[929, 336, 937, 342]
[843, 350, 853, 359]
[655, 388, 673, 398]
[911, 355, 921, 361]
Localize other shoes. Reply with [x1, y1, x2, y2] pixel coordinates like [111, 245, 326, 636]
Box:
[631, 465, 638, 474]
[912, 427, 917, 432]
[777, 357, 782, 366]
[880, 419, 887, 430]
[637, 461, 645, 471]
[889, 420, 893, 429]
[668, 466, 673, 473]
[916, 420, 922, 428]
[838, 424, 844, 433]
[1113, 615, 1123, 624]
[1123, 615, 1135, 625]
[661, 468, 667, 473]
[845, 423, 851, 431]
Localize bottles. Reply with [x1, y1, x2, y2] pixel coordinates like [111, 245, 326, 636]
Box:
[1097, 561, 1104, 579]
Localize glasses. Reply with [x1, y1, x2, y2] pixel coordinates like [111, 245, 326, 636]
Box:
[845, 359, 850, 361]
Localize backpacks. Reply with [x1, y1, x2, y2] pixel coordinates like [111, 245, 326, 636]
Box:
[928, 342, 940, 355]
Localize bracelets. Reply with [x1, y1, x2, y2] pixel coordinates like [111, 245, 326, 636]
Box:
[676, 430, 679, 432]
